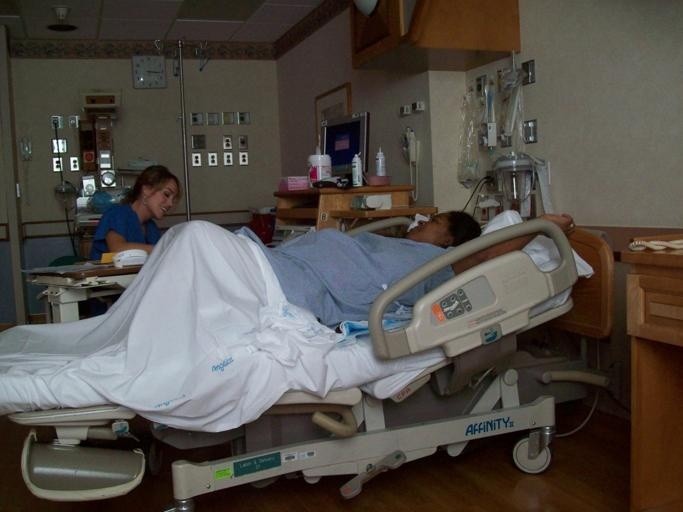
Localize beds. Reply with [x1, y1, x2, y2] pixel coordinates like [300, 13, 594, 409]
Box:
[2, 217, 612, 510]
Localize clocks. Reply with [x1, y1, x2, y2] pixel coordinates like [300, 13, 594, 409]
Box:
[131, 53, 169, 89]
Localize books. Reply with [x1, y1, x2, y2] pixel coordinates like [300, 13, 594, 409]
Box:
[16, 260, 110, 275]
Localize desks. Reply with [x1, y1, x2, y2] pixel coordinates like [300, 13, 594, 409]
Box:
[617, 227, 682, 512]
[24, 259, 145, 324]
[328, 206, 440, 233]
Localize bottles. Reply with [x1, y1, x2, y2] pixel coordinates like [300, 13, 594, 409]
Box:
[351, 152, 362, 187]
[374, 147, 386, 177]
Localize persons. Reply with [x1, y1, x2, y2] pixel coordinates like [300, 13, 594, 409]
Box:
[136, 210, 575, 354]
[88, 165, 181, 262]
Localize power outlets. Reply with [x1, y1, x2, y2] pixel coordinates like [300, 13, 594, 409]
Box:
[50, 115, 63, 129]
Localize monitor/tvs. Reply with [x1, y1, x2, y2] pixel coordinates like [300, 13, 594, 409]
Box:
[319, 111, 369, 176]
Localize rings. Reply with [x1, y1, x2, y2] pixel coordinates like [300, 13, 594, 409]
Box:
[569, 222, 573, 228]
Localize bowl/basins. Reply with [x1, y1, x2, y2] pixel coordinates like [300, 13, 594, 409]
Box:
[364, 175, 392, 186]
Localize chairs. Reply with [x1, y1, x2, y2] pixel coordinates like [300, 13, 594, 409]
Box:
[48, 254, 104, 320]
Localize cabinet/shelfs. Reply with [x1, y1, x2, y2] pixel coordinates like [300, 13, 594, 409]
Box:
[269, 182, 416, 233]
[346, 0, 524, 71]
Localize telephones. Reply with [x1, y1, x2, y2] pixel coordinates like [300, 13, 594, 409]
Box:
[21, 136, 33, 164]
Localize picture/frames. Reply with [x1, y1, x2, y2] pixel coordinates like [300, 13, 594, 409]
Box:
[315, 83, 352, 144]
